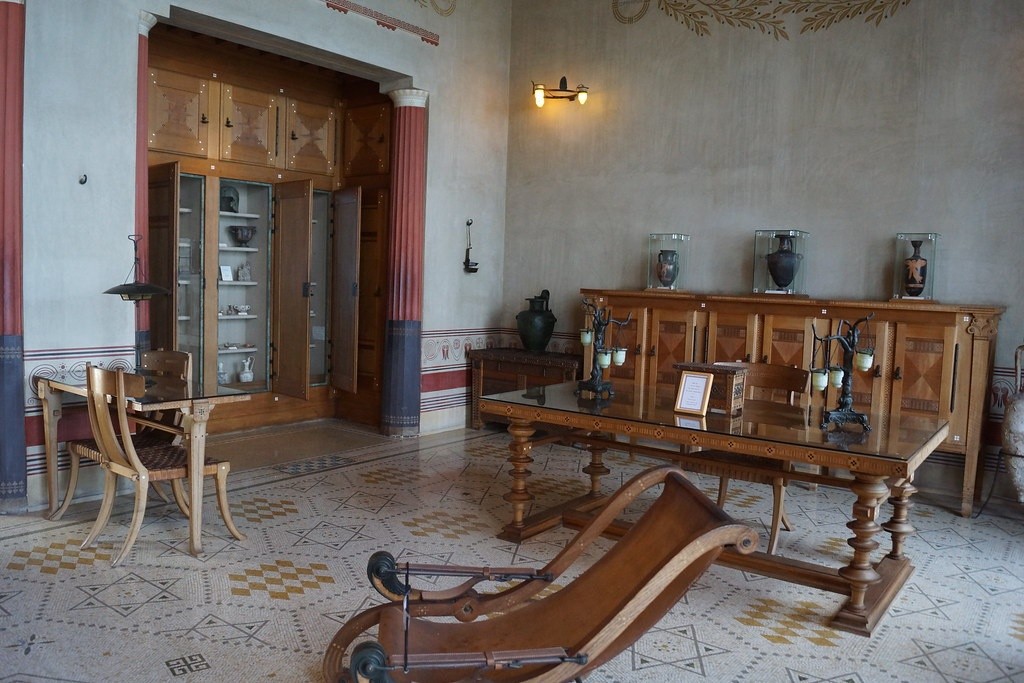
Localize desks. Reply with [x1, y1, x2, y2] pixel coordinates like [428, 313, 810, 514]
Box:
[35, 370, 253, 557]
[469, 346, 583, 445]
[477, 377, 951, 635]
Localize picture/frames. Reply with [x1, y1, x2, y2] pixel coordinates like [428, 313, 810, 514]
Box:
[674, 369, 713, 416]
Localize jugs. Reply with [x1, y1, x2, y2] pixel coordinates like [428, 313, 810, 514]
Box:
[239, 355, 255, 382]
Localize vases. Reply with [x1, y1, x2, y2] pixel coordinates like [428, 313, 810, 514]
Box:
[903, 240, 929, 295]
[655, 250, 681, 286]
[514, 289, 558, 354]
[766, 234, 802, 292]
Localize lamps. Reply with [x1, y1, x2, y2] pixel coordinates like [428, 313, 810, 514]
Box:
[805, 311, 875, 432]
[102, 234, 172, 309]
[530, 76, 589, 108]
[574, 300, 633, 397]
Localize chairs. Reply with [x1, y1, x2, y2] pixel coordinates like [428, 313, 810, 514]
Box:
[321, 465, 760, 683]
[687, 358, 810, 530]
[79, 361, 245, 567]
[48, 351, 195, 548]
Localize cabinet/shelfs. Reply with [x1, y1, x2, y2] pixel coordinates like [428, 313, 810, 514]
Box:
[579, 286, 1010, 516]
[147, 28, 391, 437]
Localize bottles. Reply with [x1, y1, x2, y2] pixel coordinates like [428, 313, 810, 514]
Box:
[656, 249, 680, 286]
[999, 345, 1024, 509]
[515, 289, 558, 352]
[764, 234, 804, 291]
[903, 240, 927, 298]
